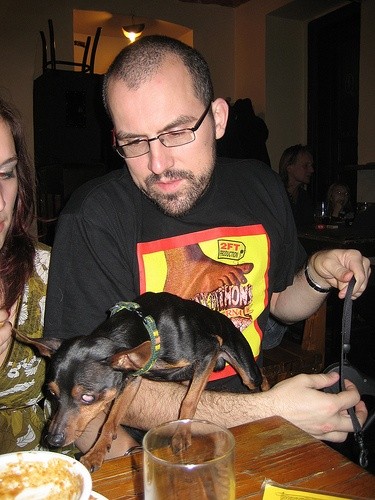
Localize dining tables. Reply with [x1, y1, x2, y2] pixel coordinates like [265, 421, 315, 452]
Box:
[87, 415, 375, 500]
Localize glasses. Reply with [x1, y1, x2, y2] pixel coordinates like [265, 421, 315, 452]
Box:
[112, 98, 213, 159]
[332, 190, 350, 196]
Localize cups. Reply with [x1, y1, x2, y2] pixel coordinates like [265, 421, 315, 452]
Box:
[316, 201, 330, 217]
[143, 419, 236, 500]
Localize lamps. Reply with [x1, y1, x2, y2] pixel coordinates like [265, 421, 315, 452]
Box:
[123, 12, 144, 43]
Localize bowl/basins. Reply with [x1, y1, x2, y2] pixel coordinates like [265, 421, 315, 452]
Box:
[0, 450, 92, 500]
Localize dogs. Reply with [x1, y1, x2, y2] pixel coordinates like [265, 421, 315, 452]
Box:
[9, 292, 271, 474]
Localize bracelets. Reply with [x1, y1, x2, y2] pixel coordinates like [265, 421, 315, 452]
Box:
[303, 260, 332, 294]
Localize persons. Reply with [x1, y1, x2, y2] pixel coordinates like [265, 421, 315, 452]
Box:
[43, 35, 371, 462]
[278, 144, 315, 235]
[326, 182, 353, 224]
[0, 98, 61, 455]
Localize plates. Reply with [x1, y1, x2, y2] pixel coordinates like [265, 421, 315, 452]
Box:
[91, 491, 109, 500]
